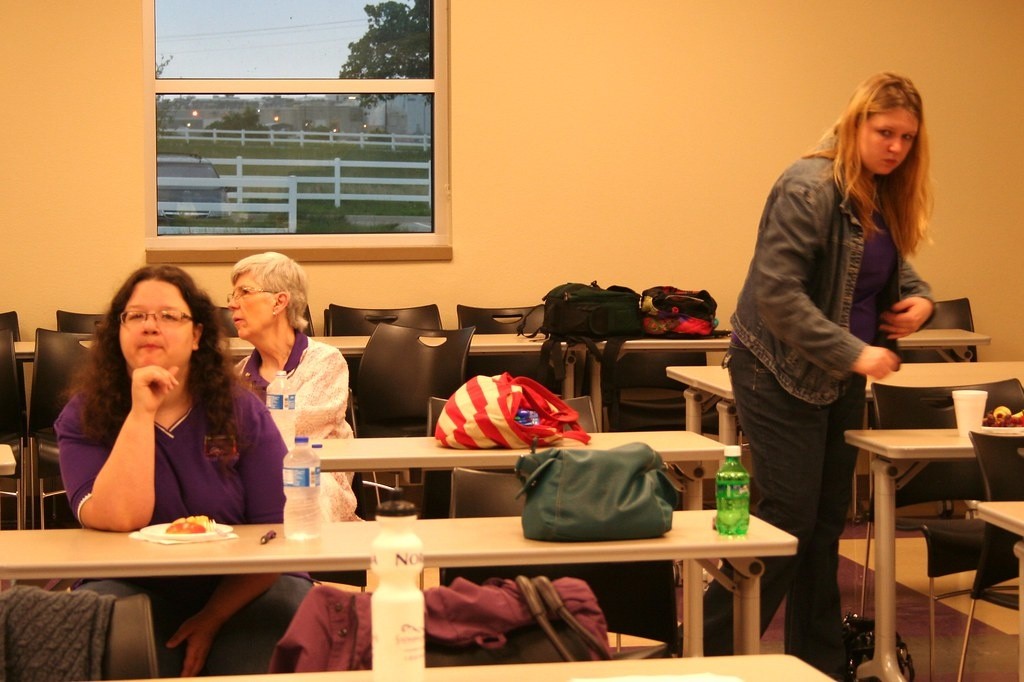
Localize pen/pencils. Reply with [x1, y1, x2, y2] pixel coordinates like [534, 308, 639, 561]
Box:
[260, 530, 277, 544]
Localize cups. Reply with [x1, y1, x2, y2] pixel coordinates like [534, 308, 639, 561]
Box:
[953, 390, 988, 437]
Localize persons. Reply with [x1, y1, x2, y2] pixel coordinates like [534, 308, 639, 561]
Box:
[53, 265, 321, 682]
[229, 252, 358, 525]
[703, 73, 936, 682]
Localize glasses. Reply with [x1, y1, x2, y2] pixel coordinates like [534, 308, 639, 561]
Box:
[227, 286, 279, 303]
[120, 311, 194, 326]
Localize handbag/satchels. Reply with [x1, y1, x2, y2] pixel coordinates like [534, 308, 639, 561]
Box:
[272, 577, 608, 674]
[515, 436, 694, 541]
[640, 286, 718, 337]
[516, 281, 639, 428]
[842, 613, 914, 682]
[435, 373, 591, 449]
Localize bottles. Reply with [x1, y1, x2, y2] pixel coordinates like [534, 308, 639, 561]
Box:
[265, 371, 295, 449]
[282, 438, 323, 541]
[370, 487, 424, 682]
[716, 445, 749, 535]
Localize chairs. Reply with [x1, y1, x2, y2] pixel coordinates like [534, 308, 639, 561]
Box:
[854, 297, 977, 522]
[0, 303, 739, 520]
[860, 377, 1024, 682]
[956, 432, 1024, 682]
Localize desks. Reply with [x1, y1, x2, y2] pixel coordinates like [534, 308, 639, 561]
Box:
[0, 325, 1024, 682]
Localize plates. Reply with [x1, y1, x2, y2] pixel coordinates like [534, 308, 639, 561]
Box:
[141, 523, 232, 541]
[982, 426, 1024, 433]
